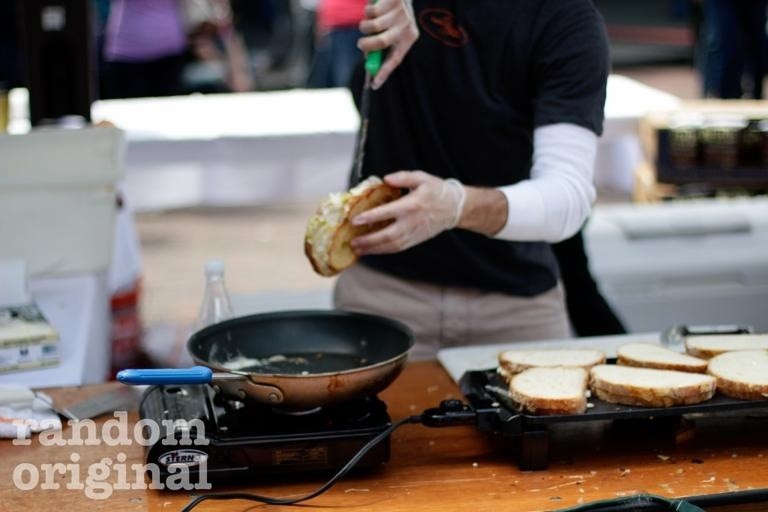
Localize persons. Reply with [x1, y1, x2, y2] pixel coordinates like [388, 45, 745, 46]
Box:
[693, 0, 768, 98]
[103, 0, 373, 97]
[333, 0, 611, 364]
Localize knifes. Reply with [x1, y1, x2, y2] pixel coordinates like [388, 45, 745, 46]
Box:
[350, 45, 382, 189]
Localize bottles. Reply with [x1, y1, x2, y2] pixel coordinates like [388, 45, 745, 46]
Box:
[196, 261, 234, 332]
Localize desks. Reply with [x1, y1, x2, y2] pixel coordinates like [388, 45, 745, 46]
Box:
[2, 342, 766, 509]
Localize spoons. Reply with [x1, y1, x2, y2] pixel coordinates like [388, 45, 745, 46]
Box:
[116, 308, 417, 409]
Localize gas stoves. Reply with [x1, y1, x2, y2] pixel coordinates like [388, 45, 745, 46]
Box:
[137, 369, 394, 490]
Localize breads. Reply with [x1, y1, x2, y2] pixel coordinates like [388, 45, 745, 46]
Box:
[497, 334, 768, 414]
[304, 176, 399, 277]
